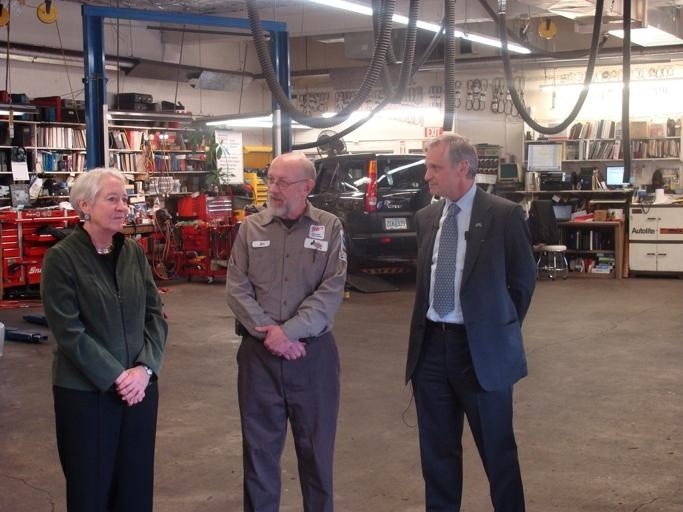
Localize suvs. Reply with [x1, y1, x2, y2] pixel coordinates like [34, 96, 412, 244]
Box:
[309, 155, 432, 270]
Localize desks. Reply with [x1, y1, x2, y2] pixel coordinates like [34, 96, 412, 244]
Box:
[519, 189, 629, 279]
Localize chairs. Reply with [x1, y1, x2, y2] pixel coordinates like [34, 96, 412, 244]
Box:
[526, 198, 564, 277]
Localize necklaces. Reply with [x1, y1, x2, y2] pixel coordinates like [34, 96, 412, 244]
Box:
[96, 245, 112, 255]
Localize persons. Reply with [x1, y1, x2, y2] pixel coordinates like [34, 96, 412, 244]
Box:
[222, 153, 347, 510]
[38, 168, 167, 512]
[403, 132, 537, 511]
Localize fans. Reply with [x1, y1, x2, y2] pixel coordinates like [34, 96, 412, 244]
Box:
[314, 130, 347, 159]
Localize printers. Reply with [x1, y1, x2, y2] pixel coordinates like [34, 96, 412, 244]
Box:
[541, 171, 576, 190]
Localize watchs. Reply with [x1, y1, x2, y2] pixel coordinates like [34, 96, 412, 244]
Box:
[143, 364, 152, 377]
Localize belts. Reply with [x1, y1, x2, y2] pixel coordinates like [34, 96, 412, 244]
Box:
[426, 319, 466, 330]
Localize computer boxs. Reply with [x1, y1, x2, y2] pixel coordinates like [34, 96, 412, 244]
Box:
[581, 167, 599, 190]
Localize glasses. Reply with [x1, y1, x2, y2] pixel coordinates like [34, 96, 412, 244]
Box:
[260, 175, 312, 192]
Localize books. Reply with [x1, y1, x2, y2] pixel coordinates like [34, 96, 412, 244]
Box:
[566, 167, 614, 274]
[568, 117, 680, 160]
[33, 125, 209, 199]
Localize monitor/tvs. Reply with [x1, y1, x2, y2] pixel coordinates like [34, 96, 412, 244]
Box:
[606, 166, 625, 189]
[500, 163, 519, 183]
[526, 143, 563, 173]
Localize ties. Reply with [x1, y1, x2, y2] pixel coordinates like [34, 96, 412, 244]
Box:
[431, 205, 463, 319]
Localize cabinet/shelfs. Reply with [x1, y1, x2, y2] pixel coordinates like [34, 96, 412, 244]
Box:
[628, 205, 682, 277]
[0, 118, 217, 239]
[523, 137, 683, 162]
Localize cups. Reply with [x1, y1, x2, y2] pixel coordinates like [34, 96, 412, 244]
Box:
[654, 189, 664, 201]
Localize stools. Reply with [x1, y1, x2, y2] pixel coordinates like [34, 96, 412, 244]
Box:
[536, 244, 568, 279]
